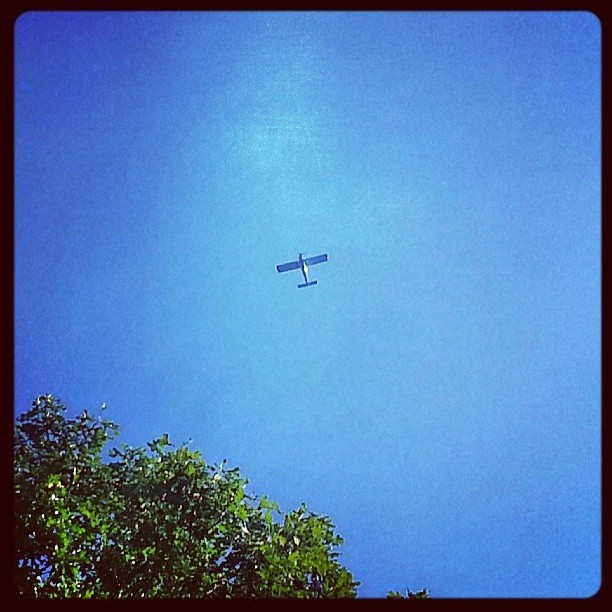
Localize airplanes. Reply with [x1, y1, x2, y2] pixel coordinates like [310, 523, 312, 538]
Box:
[277, 253, 327, 288]
